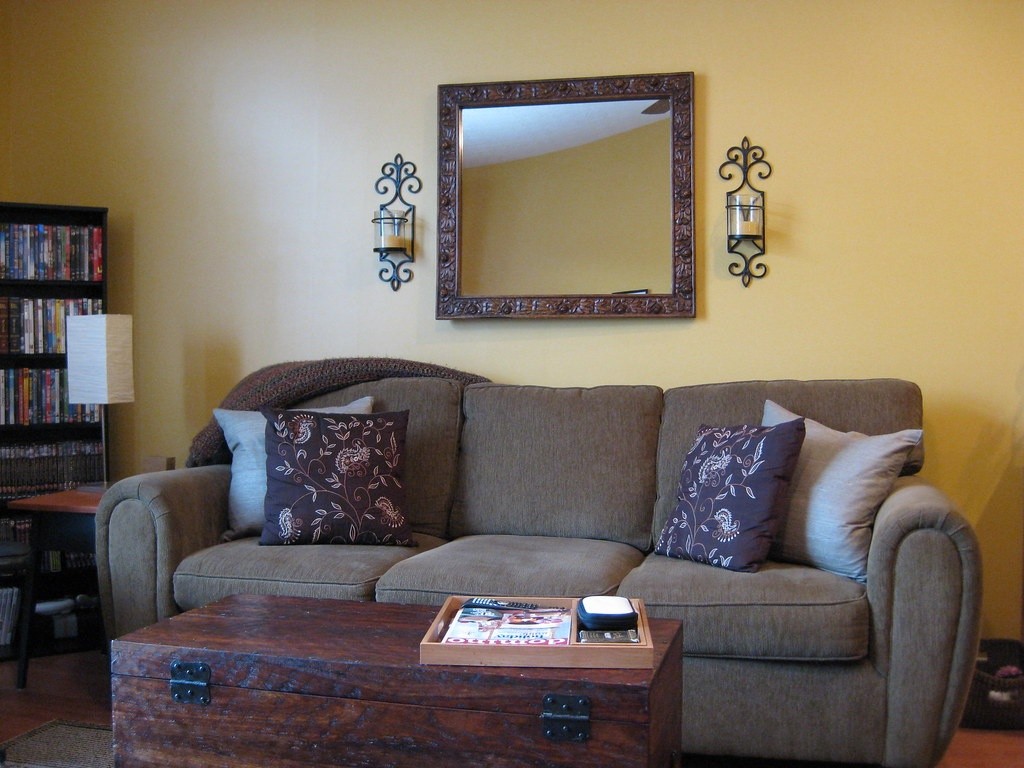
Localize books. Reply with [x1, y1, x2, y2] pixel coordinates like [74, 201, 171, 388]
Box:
[441, 607, 571, 647]
[0, 223, 104, 645]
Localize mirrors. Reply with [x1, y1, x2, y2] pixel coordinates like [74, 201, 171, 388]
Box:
[437, 71, 697, 319]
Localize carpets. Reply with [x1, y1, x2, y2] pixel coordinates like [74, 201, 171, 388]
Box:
[0, 717, 117, 767]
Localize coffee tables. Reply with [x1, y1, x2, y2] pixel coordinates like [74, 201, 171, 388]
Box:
[109, 596, 685, 767]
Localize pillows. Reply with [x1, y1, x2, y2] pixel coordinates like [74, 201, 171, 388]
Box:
[654, 414, 807, 572]
[759, 399, 924, 587]
[258, 404, 422, 550]
[213, 396, 371, 542]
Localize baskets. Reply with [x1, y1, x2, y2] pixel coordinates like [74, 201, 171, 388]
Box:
[960, 660, 1024, 729]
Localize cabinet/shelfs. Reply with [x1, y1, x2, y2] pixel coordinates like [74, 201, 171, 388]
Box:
[0, 203, 108, 661]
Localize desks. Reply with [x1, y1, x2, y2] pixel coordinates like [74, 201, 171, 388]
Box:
[7, 490, 108, 688]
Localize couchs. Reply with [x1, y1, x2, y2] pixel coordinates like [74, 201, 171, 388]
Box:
[94, 376, 983, 768]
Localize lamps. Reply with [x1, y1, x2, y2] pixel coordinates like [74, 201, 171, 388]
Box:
[718, 136, 772, 287]
[371, 153, 421, 291]
[66, 315, 135, 493]
